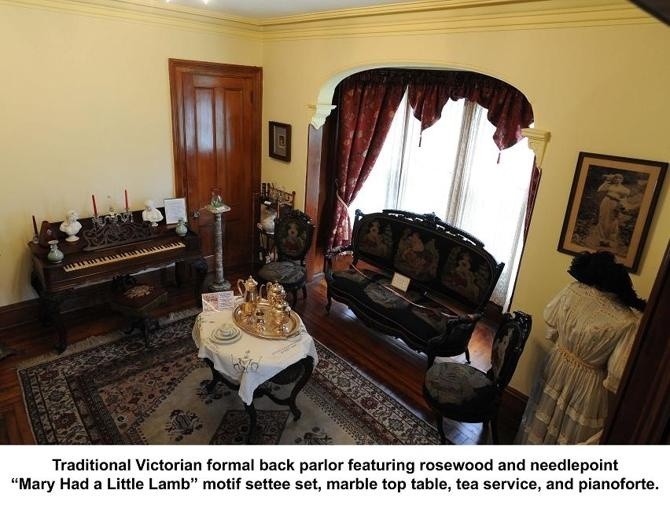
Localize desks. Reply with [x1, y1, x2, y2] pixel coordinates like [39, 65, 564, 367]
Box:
[190, 296, 315, 444]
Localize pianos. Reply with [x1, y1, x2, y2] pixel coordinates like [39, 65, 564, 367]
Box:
[27, 203, 209, 354]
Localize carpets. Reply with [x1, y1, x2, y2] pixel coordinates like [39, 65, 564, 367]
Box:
[15, 307, 452, 444]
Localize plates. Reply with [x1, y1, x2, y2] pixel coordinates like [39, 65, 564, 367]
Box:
[208, 327, 240, 344]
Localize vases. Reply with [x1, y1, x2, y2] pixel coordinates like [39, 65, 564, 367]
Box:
[47, 239, 64, 264]
[176, 217, 188, 237]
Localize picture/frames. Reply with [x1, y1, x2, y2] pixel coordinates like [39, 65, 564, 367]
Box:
[268, 120, 291, 162]
[556, 150, 670, 275]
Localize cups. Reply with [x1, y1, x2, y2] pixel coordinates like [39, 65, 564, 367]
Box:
[220, 324, 233, 335]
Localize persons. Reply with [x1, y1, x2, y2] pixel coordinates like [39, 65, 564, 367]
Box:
[60, 210, 82, 236]
[596, 170, 630, 245]
[142, 201, 163, 221]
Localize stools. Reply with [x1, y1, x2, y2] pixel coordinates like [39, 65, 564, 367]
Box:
[108, 279, 169, 349]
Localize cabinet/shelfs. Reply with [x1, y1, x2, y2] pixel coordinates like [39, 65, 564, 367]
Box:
[255, 182, 296, 266]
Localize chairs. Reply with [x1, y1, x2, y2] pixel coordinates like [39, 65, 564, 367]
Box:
[256, 209, 314, 309]
[422, 310, 533, 444]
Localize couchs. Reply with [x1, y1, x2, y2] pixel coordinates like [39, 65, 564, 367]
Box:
[323, 208, 505, 370]
